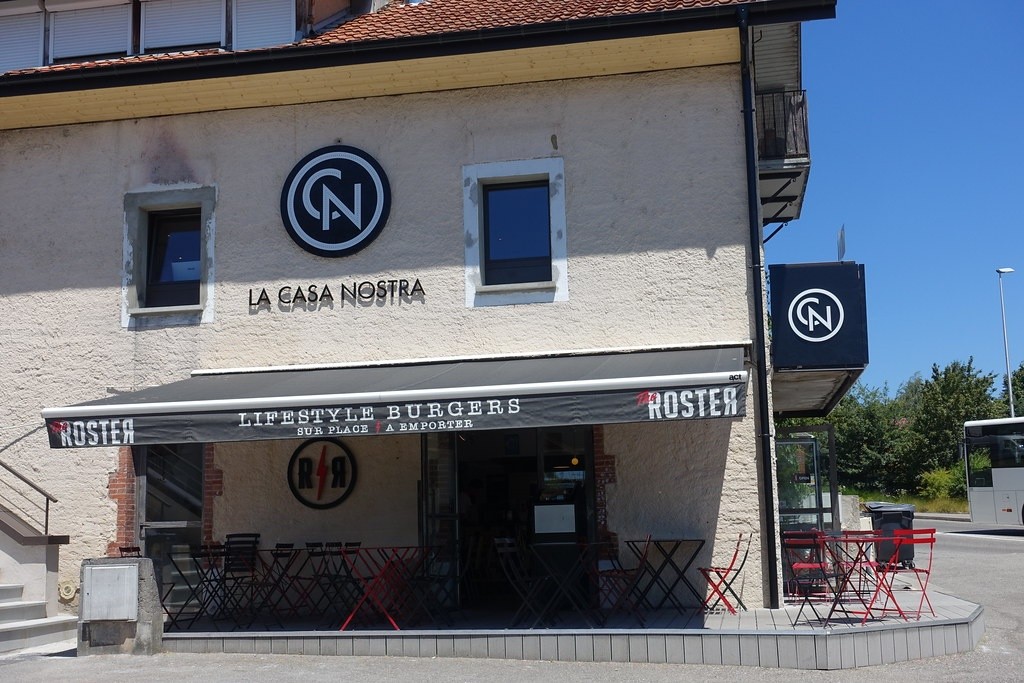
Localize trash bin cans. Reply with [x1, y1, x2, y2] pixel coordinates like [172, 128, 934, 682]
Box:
[863, 502, 916, 569]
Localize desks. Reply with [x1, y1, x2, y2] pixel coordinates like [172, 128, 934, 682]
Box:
[167, 552, 249, 631]
[525, 541, 611, 630]
[811, 536, 909, 625]
[242, 547, 316, 624]
[625, 539, 705, 612]
[338, 543, 449, 631]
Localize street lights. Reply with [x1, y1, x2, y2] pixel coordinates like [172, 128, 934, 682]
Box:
[997, 267, 1017, 418]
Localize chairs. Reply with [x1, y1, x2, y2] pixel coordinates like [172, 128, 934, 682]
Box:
[394, 534, 650, 629]
[119, 532, 385, 632]
[782, 528, 937, 628]
[697, 533, 753, 616]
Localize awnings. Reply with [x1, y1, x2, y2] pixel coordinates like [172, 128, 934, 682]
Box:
[41, 341, 754, 449]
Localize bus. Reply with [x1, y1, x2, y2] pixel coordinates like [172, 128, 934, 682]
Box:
[958, 417, 1024, 531]
